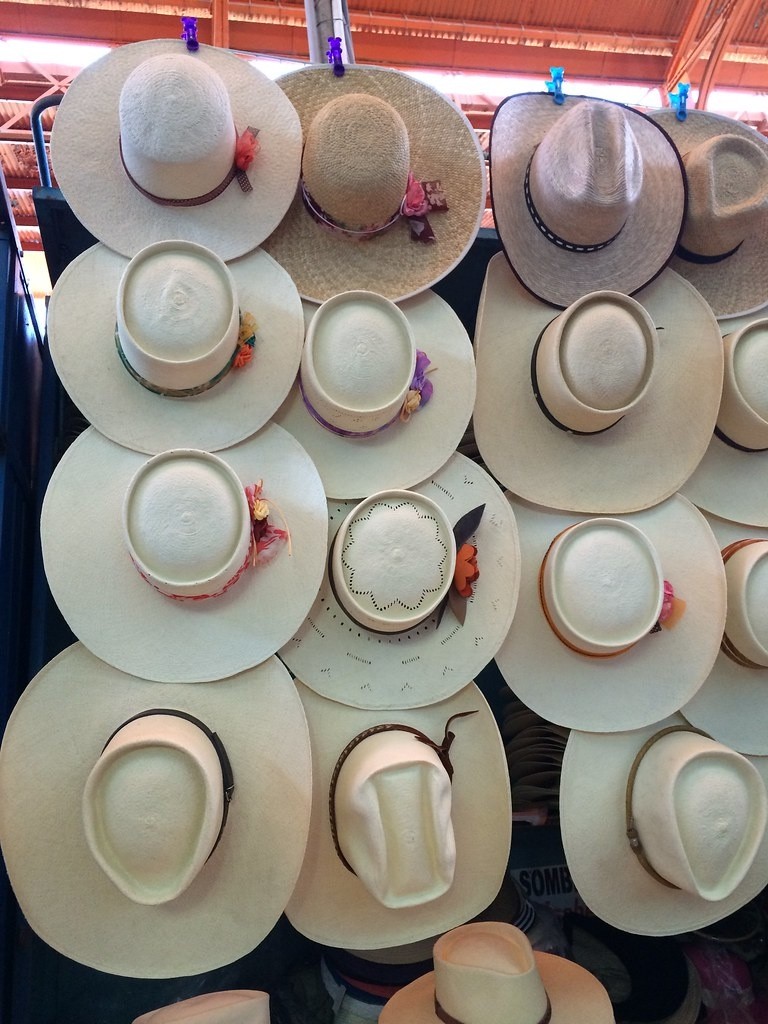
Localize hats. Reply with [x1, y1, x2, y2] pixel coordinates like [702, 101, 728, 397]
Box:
[645, 109, 768, 319]
[321, 876, 575, 1024]
[377, 922, 616, 1024]
[677, 304, 768, 528]
[40, 421, 328, 684]
[49, 39, 301, 264]
[283, 679, 513, 950]
[560, 709, 768, 937]
[258, 63, 487, 304]
[129, 989, 270, 1024]
[490, 480, 727, 732]
[275, 451, 521, 710]
[269, 289, 477, 500]
[0, 641, 313, 979]
[47, 241, 305, 456]
[489, 92, 686, 311]
[677, 504, 768, 756]
[472, 252, 724, 514]
